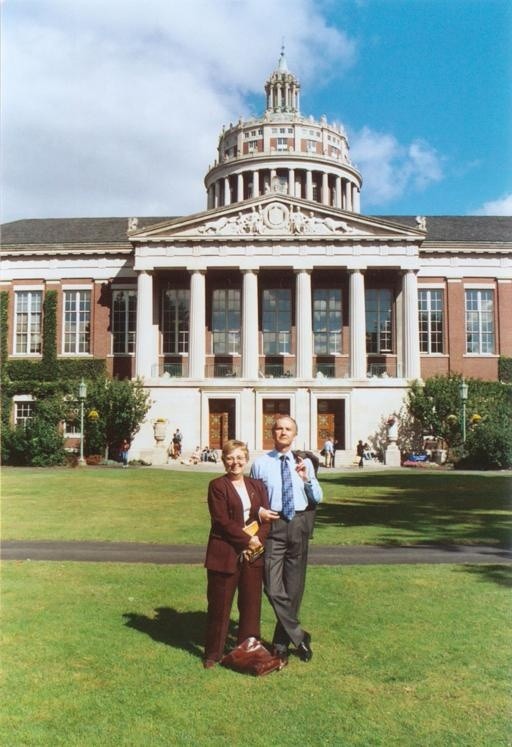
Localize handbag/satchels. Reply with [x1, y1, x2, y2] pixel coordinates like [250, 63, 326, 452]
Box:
[320, 449, 326, 458]
[220, 635, 283, 677]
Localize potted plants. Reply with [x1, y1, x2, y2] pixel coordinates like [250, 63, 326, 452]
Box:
[385, 419, 399, 445]
[154, 418, 169, 444]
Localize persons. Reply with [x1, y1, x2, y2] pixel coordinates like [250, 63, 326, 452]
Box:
[363, 443, 372, 460]
[356, 440, 364, 467]
[201, 440, 271, 669]
[248, 416, 323, 669]
[330, 440, 339, 467]
[324, 436, 333, 468]
[166, 427, 219, 464]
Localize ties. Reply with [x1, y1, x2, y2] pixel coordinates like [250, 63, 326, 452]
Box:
[279, 456, 295, 521]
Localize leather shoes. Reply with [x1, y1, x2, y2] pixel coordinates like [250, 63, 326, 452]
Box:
[204, 659, 216, 671]
[271, 646, 287, 661]
[297, 631, 312, 662]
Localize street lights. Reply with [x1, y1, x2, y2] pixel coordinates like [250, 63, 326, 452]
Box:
[458, 379, 469, 443]
[75, 376, 87, 467]
